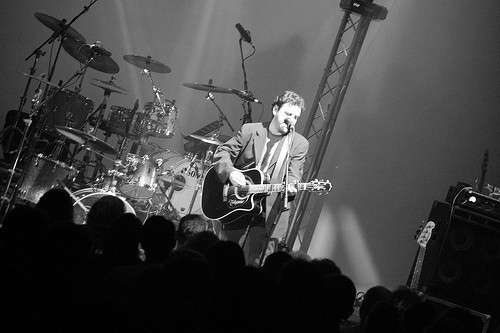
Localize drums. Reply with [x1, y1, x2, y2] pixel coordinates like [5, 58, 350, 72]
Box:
[106, 105, 144, 140]
[70, 187, 138, 224]
[17, 152, 80, 204]
[142, 102, 179, 139]
[103, 159, 122, 196]
[118, 152, 158, 199]
[151, 156, 210, 217]
[35, 88, 95, 139]
[183, 134, 223, 164]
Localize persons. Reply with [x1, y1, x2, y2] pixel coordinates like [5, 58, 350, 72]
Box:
[213, 92, 310, 265]
[0, 189, 356, 333]
[353, 285, 446, 333]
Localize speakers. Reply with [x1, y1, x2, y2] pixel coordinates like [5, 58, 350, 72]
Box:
[410, 198, 500, 319]
[404, 291, 500, 333]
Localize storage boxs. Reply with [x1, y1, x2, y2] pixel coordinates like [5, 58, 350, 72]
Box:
[414, 291, 492, 333]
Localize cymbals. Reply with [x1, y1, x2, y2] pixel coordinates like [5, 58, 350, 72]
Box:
[34, 12, 87, 43]
[16, 71, 61, 89]
[122, 55, 173, 73]
[55, 125, 120, 156]
[89, 77, 131, 95]
[217, 135, 233, 143]
[182, 82, 234, 94]
[60, 38, 120, 74]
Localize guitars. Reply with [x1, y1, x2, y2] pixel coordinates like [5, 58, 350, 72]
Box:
[200, 165, 333, 220]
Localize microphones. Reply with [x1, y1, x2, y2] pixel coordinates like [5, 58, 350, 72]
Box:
[232, 88, 263, 105]
[90, 44, 112, 56]
[235, 23, 255, 48]
[284, 119, 295, 130]
